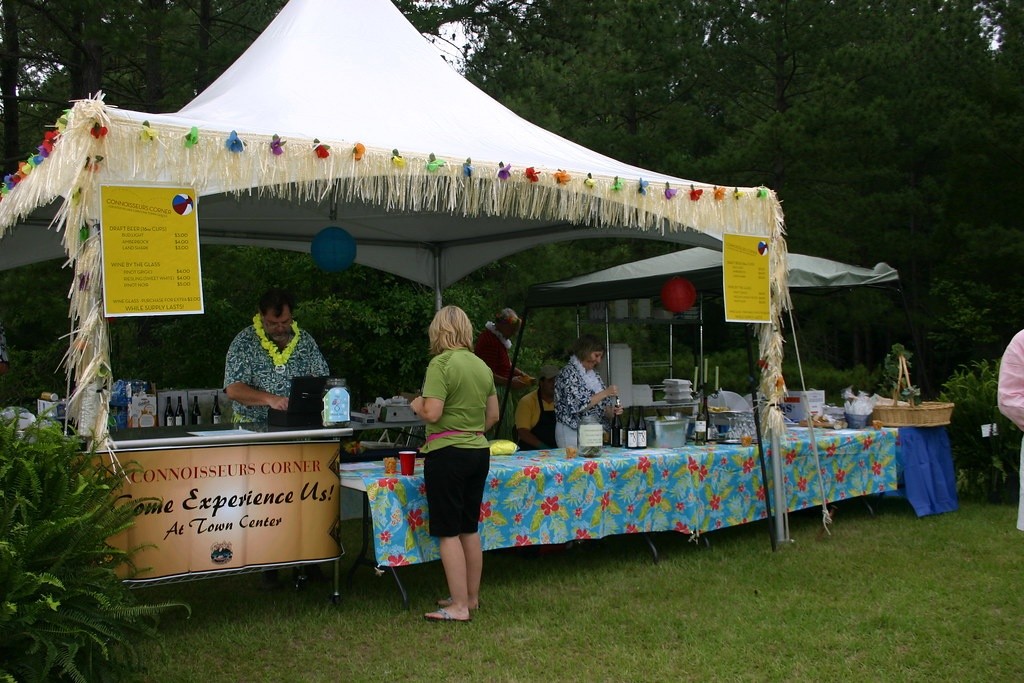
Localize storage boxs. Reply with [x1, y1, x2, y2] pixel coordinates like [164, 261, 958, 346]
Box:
[783, 390, 825, 421]
[662, 379, 693, 403]
[643, 416, 687, 448]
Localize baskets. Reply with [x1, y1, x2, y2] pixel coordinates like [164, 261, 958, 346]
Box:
[871, 352, 955, 425]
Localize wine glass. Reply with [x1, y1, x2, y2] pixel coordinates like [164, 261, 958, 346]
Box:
[708, 410, 757, 440]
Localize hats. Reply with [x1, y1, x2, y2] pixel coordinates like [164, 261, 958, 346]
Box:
[538, 364, 560, 379]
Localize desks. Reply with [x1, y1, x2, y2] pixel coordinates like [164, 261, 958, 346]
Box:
[344, 418, 425, 455]
[664, 423, 899, 547]
[336, 444, 690, 604]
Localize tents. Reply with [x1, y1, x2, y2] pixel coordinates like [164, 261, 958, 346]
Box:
[0, 0, 791, 550]
[493, 244, 918, 449]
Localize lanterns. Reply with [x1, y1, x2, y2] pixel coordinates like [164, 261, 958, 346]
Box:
[660, 276, 697, 319]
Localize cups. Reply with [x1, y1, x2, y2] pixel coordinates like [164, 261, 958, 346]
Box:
[381, 451, 397, 476]
[872, 419, 884, 431]
[740, 435, 752, 446]
[399, 451, 417, 475]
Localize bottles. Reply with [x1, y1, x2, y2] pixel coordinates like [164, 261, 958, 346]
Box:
[637, 406, 648, 449]
[627, 406, 638, 449]
[695, 403, 707, 446]
[107, 378, 148, 432]
[577, 414, 604, 457]
[164, 396, 174, 427]
[320, 378, 351, 427]
[610, 405, 623, 447]
[211, 395, 222, 425]
[174, 396, 185, 426]
[192, 396, 202, 425]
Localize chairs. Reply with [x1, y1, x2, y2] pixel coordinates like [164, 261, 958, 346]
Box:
[360, 391, 420, 458]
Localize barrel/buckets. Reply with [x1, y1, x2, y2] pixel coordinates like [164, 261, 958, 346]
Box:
[844, 411, 873, 430]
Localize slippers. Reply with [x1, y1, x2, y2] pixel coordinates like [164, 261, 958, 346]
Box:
[424, 608, 471, 622]
[434, 597, 479, 611]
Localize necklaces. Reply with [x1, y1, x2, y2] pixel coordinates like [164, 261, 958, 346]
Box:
[484, 321, 512, 348]
[568, 353, 602, 395]
[253, 312, 301, 366]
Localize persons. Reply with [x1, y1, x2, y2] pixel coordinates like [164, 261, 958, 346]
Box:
[552, 335, 623, 448]
[515, 363, 561, 450]
[997, 329, 1024, 530]
[409, 305, 499, 624]
[477, 308, 537, 441]
[223, 288, 330, 593]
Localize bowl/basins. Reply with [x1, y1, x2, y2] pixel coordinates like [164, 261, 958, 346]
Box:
[655, 421, 686, 448]
[643, 415, 689, 448]
[662, 379, 692, 404]
